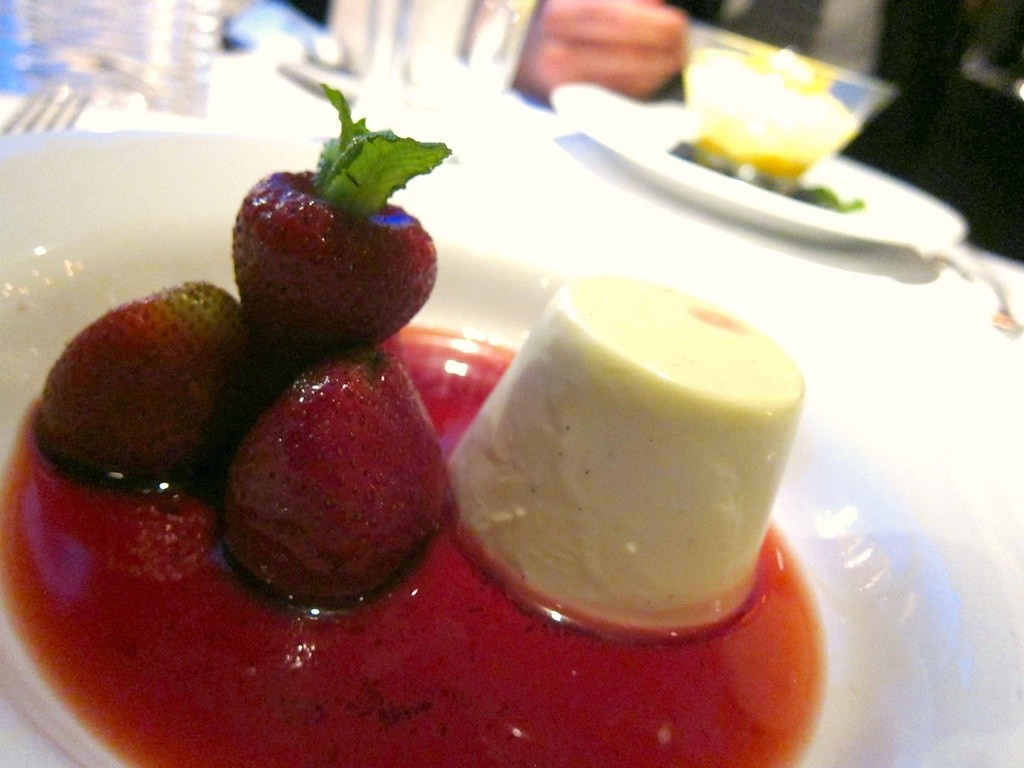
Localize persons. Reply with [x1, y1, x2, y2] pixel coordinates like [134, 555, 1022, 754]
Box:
[220, 0, 965, 185]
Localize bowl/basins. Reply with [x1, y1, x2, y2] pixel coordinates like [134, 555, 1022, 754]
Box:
[683, 20, 900, 184]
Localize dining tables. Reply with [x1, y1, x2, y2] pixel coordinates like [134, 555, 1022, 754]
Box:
[0, 30, 1024, 768]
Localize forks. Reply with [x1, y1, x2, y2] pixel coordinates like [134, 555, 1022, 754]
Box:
[0, 86, 90, 133]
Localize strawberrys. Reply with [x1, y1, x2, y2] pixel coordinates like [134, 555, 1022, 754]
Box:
[29, 278, 253, 494]
[222, 343, 452, 614]
[222, 78, 446, 356]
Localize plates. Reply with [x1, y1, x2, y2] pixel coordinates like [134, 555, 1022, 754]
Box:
[0, 123, 1024, 767]
[551, 84, 965, 252]
